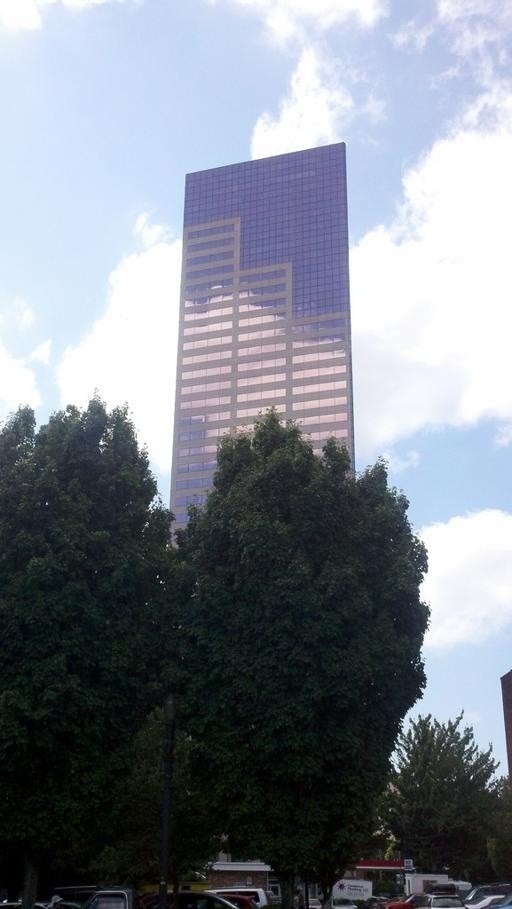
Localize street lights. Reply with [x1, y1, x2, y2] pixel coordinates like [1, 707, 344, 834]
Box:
[159, 695, 175, 909]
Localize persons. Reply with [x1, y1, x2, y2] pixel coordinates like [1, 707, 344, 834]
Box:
[46, 894, 64, 908]
[296, 887, 306, 909]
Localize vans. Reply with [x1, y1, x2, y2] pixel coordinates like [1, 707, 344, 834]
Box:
[0, 886, 268, 909]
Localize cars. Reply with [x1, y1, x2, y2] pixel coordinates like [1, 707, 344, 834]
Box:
[305, 895, 358, 909]
[367, 880, 511, 909]
